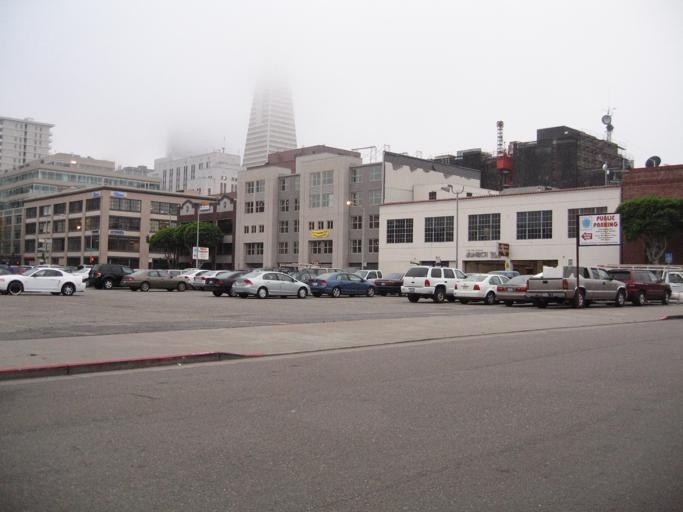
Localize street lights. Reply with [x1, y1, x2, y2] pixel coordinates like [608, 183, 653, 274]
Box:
[439, 184, 465, 268]
[346, 200, 366, 271]
[76, 226, 92, 264]
